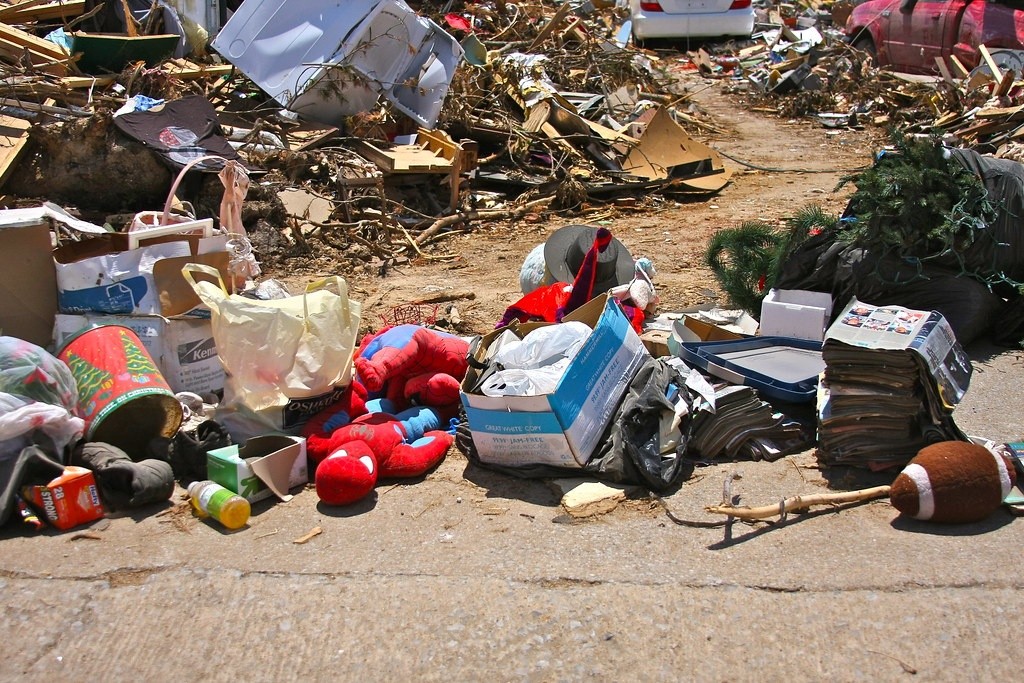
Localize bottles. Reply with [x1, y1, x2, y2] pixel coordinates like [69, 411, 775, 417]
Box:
[186, 481, 251, 529]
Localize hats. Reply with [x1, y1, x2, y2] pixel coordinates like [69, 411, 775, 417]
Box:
[544, 225, 634, 295]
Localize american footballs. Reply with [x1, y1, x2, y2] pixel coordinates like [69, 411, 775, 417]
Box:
[890, 441, 1016, 524]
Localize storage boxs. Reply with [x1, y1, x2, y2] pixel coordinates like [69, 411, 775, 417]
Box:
[53, 232, 229, 394]
[760, 287, 832, 340]
[21, 466, 104, 532]
[459, 291, 649, 468]
[206, 433, 308, 503]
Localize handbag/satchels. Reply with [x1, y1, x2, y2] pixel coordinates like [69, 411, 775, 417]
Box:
[481, 320, 595, 396]
[182, 263, 362, 401]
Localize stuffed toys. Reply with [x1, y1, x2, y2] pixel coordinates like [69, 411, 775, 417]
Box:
[299, 323, 468, 507]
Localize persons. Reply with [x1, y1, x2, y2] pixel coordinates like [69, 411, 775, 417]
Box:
[895, 326, 907, 333]
[847, 316, 859, 325]
[899, 312, 913, 322]
[855, 307, 868, 315]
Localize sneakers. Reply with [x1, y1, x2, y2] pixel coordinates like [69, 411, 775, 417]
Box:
[68, 441, 174, 509]
[168, 420, 233, 479]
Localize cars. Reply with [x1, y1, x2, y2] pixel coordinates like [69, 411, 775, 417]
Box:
[842, 0, 1024, 73]
[625, 0, 757, 49]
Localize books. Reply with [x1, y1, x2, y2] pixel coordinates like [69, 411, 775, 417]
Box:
[815, 294, 975, 473]
[665, 356, 811, 464]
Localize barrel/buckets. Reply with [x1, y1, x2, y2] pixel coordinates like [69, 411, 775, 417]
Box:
[55, 320, 183, 462]
